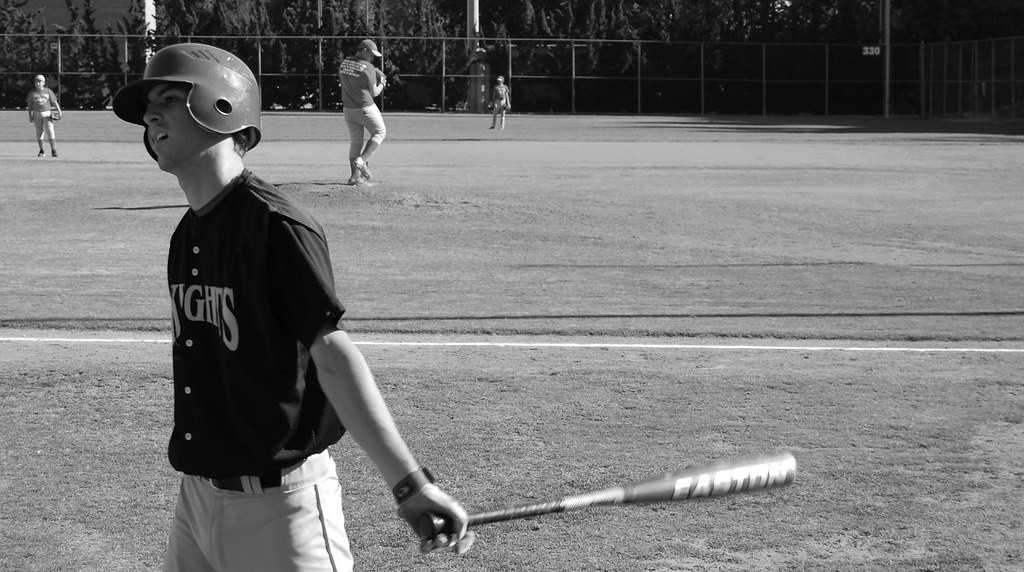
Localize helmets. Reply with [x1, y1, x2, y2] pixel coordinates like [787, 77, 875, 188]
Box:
[113, 43, 261, 161]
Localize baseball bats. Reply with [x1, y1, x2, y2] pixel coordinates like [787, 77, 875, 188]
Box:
[415, 452, 797, 538]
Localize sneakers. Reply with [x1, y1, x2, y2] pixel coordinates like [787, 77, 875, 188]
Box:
[352, 156, 371, 179]
[349, 177, 372, 187]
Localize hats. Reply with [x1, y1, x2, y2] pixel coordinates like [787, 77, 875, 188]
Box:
[497, 76, 504, 81]
[359, 39, 382, 57]
[34, 75, 45, 82]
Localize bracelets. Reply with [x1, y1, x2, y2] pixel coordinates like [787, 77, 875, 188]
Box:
[392, 465, 436, 506]
[378, 81, 384, 88]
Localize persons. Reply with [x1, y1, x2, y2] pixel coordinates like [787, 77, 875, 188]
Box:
[111, 42, 474, 572]
[28, 74, 62, 158]
[489, 74, 512, 129]
[339, 39, 388, 187]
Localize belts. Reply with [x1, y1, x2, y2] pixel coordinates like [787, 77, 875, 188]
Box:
[201, 469, 281, 492]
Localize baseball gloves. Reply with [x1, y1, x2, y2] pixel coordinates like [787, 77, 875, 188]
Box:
[51, 113, 61, 120]
[375, 68, 387, 87]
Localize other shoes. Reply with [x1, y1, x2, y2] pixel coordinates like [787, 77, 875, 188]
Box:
[38, 149, 45, 157]
[52, 151, 57, 156]
